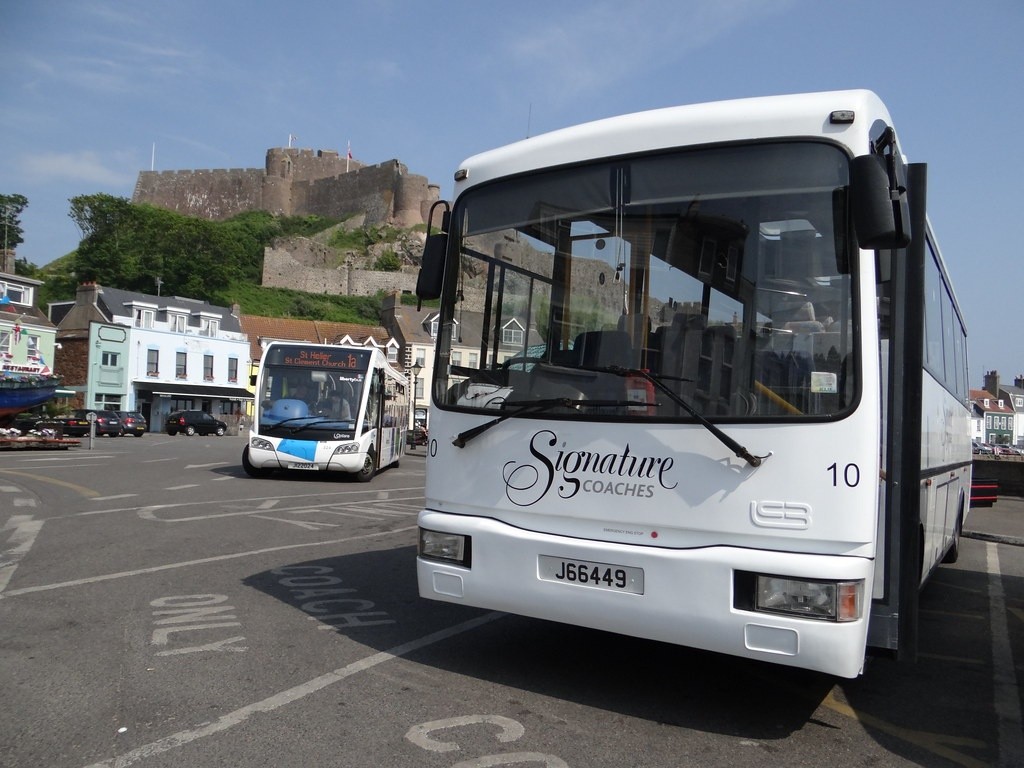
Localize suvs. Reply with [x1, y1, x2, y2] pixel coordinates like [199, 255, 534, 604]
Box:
[165, 410, 228, 436]
[115, 410, 147, 437]
[84, 409, 123, 438]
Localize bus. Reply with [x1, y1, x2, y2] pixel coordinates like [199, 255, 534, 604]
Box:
[243, 340, 411, 483]
[414, 87, 974, 680]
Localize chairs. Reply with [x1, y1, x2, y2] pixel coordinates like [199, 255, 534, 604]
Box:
[571, 301, 827, 415]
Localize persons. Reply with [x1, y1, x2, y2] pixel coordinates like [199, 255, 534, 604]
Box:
[324, 389, 351, 420]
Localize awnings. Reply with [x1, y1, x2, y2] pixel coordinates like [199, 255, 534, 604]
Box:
[132, 379, 256, 404]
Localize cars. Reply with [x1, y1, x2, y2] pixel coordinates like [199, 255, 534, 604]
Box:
[971, 438, 1024, 455]
[34, 411, 90, 438]
[404, 429, 429, 446]
